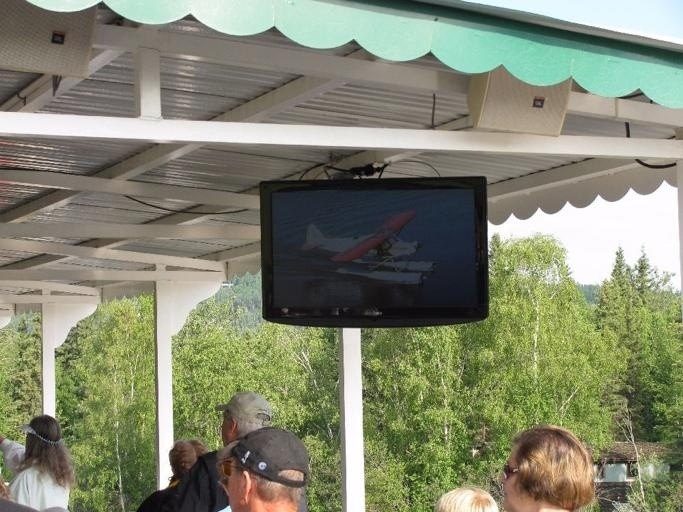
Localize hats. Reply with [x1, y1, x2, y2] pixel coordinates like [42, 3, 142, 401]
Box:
[16, 421, 61, 447]
[215, 425, 307, 485]
[210, 391, 270, 427]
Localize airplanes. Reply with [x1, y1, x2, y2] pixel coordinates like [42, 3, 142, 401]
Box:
[303, 211, 436, 285]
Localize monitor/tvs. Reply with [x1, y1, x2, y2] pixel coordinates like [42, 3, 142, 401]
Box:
[259, 175, 490, 329]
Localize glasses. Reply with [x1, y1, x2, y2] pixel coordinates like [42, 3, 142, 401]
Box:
[218, 458, 246, 479]
[499, 463, 519, 482]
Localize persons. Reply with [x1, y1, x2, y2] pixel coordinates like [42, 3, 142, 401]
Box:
[0, 474, 68, 512]
[494, 420, 595, 510]
[212, 422, 308, 512]
[167, 437, 207, 485]
[134, 390, 307, 512]
[431, 484, 500, 512]
[0, 412, 77, 508]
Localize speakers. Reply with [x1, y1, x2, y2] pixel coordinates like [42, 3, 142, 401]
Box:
[467, 67, 572, 138]
[0, 0, 98, 78]
